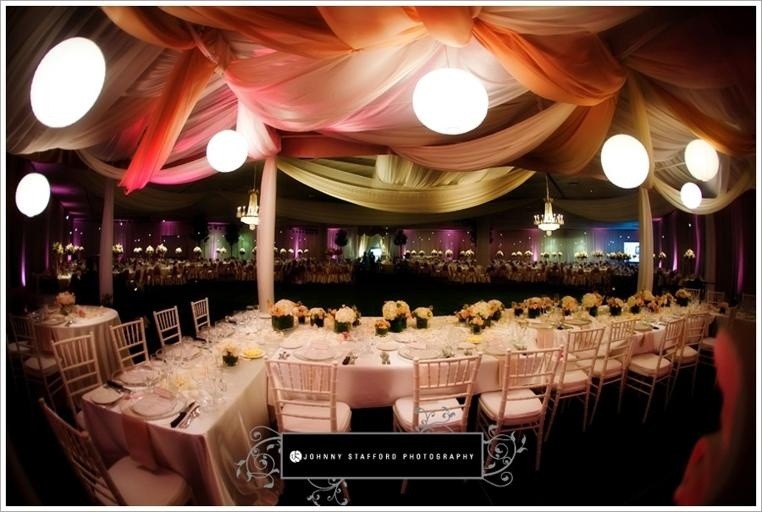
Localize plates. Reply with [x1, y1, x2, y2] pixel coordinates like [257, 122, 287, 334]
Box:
[239, 349, 265, 358]
[376, 342, 398, 351]
[398, 344, 444, 360]
[111, 365, 167, 386]
[90, 387, 124, 404]
[395, 335, 417, 343]
[280, 342, 303, 349]
[456, 342, 476, 356]
[119, 390, 185, 421]
[561, 318, 592, 325]
[635, 323, 654, 331]
[129, 388, 178, 417]
[294, 343, 341, 361]
[41, 315, 65, 325]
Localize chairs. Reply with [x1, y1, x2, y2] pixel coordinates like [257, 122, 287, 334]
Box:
[49, 331, 105, 416]
[35, 395, 196, 506]
[9, 314, 69, 405]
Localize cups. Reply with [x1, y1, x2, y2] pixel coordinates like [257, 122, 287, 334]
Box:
[211, 368, 227, 406]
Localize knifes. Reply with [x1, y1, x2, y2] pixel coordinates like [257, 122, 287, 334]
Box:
[170, 401, 197, 427]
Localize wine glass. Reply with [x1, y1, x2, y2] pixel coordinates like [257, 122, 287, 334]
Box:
[233, 311, 243, 329]
[128, 305, 265, 410]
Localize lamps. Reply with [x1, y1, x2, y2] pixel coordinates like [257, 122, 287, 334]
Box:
[236, 162, 261, 230]
[533, 173, 566, 237]
